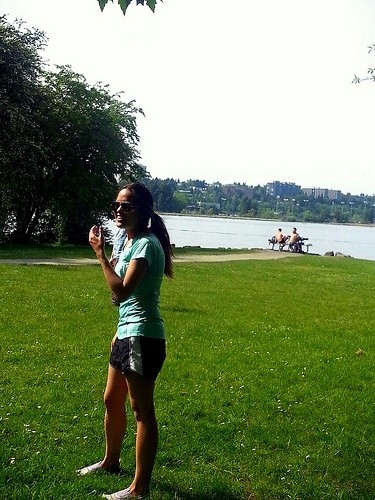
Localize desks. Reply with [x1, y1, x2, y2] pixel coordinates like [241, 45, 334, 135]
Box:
[272, 236, 308, 249]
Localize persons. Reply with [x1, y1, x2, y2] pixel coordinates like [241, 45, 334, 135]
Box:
[275, 227, 300, 253]
[76, 182, 173, 500]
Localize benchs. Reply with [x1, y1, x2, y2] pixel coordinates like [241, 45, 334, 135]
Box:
[270, 242, 304, 252]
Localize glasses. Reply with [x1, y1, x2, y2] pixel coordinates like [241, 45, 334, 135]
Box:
[112, 201, 141, 213]
